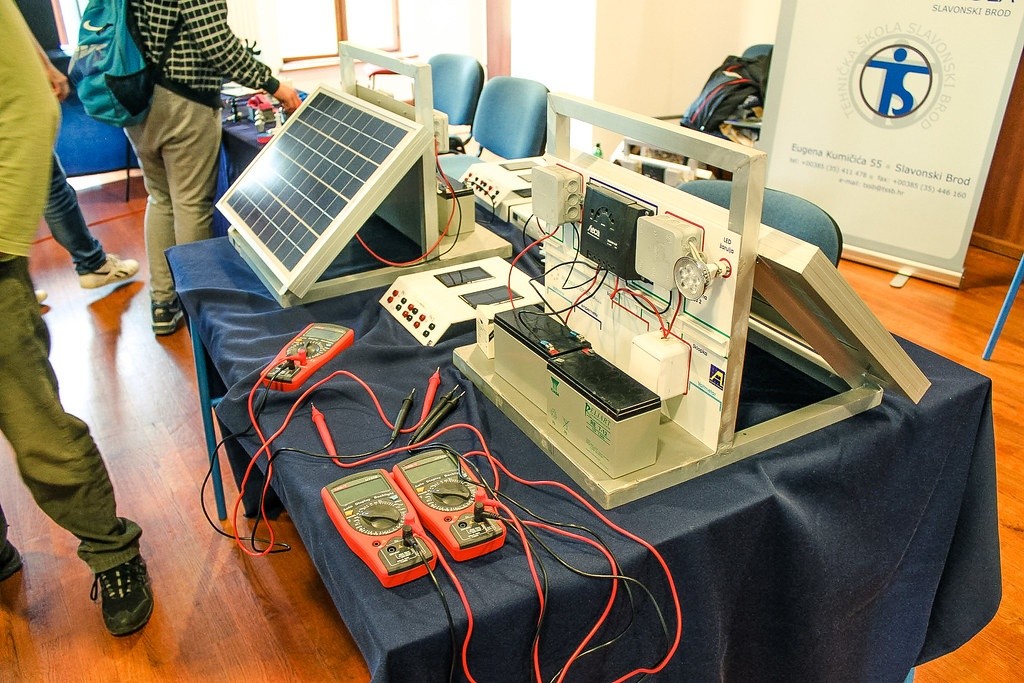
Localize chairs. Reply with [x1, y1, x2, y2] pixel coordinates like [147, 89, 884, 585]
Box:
[427, 52, 484, 147]
[676, 181, 843, 327]
[471, 76, 550, 160]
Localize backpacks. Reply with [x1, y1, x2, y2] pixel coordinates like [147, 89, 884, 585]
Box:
[67, 0, 184, 128]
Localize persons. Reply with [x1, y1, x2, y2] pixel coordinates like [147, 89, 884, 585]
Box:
[17, 10, 140, 304]
[0, 0, 154, 635]
[122, 0, 302, 335]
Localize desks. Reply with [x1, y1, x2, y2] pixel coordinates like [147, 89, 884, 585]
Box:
[164, 89, 1002, 683]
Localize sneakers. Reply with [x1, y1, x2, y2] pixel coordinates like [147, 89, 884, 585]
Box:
[152, 298, 184, 334]
[90, 554, 154, 635]
[0, 539, 22, 581]
[34, 290, 47, 304]
[79, 253, 139, 289]
